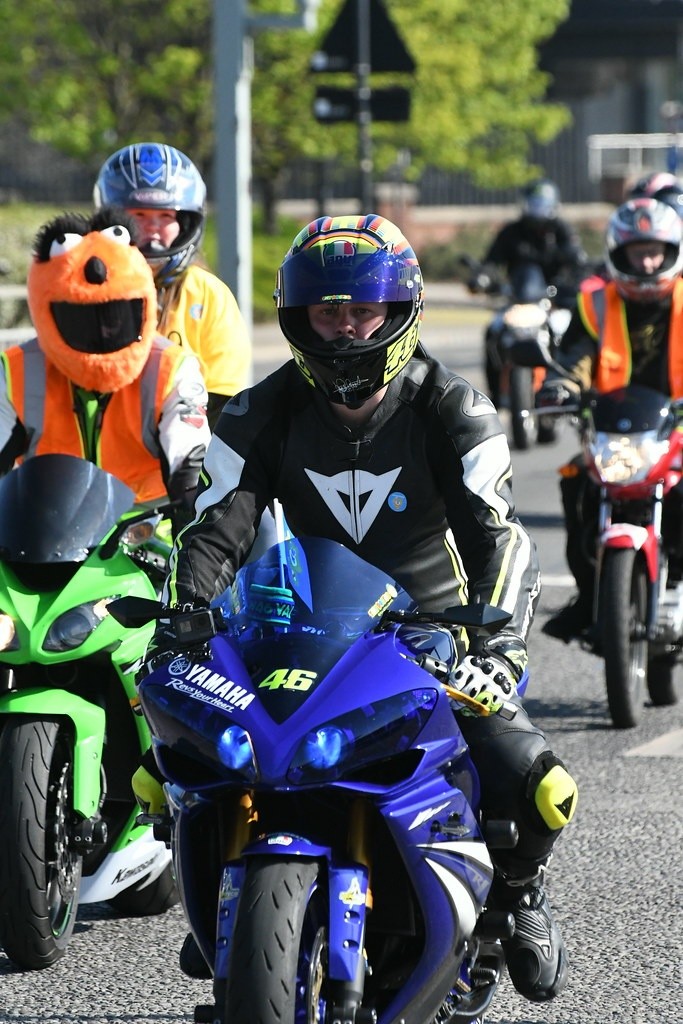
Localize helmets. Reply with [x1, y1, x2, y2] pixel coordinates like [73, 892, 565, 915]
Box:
[48, 298, 143, 355]
[603, 198, 681, 298]
[275, 215, 426, 402]
[93, 142, 207, 287]
[517, 178, 559, 221]
[627, 169, 683, 217]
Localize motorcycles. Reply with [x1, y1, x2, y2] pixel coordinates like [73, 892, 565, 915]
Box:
[104, 523, 525, 1024]
[0, 452, 180, 967]
[456, 244, 588, 447]
[517, 329, 683, 727]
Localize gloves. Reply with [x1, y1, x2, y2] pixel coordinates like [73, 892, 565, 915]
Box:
[445, 654, 517, 718]
[536, 386, 574, 410]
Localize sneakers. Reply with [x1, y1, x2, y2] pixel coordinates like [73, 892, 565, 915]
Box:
[498, 876, 570, 1001]
[653, 583, 683, 645]
[539, 597, 593, 642]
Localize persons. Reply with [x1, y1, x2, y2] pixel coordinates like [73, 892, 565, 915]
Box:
[468, 177, 583, 361]
[0, 221, 213, 575]
[532, 193, 683, 654]
[89, 138, 249, 437]
[143, 210, 576, 1006]
